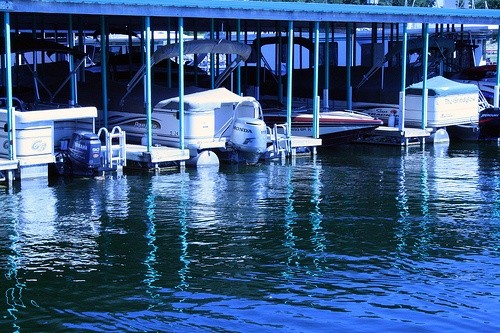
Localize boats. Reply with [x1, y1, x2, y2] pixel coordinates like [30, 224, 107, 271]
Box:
[207, 25, 495, 75]
[0, 97, 98, 177]
[356, 62, 499, 125]
[264, 102, 383, 138]
[16, 36, 164, 75]
[46, 38, 267, 171]
